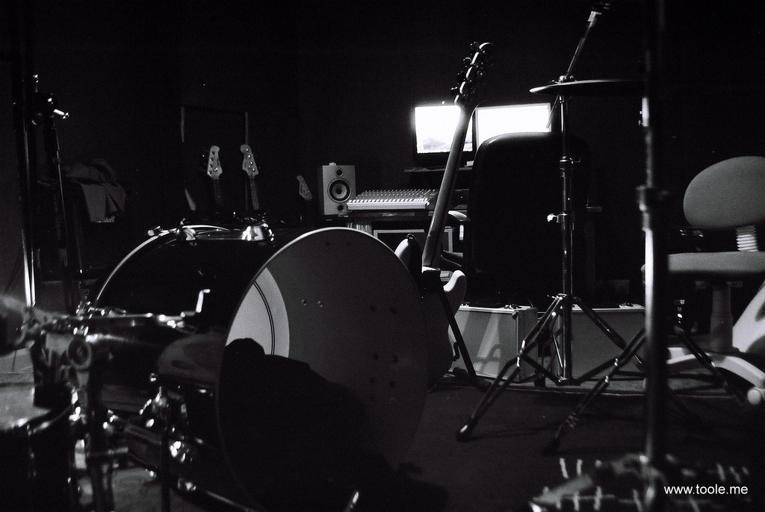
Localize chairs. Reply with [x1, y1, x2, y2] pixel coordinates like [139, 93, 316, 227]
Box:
[435, 127, 765, 405]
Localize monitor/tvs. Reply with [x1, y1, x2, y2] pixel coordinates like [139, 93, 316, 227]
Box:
[474, 101, 555, 152]
[407, 97, 474, 169]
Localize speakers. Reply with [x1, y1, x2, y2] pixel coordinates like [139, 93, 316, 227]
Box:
[317, 160, 357, 217]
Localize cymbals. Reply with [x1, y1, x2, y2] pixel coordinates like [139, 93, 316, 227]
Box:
[529, 78, 717, 102]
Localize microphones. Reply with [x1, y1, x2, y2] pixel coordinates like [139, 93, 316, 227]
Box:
[452, 38, 498, 103]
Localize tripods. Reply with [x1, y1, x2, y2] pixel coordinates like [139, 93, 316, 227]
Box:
[551, 90, 761, 456]
[457, 90, 723, 444]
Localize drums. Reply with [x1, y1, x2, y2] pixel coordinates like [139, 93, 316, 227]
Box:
[71, 226, 429, 509]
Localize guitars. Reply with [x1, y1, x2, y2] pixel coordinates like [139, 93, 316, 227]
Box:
[241, 143, 262, 212]
[206, 146, 225, 224]
[392, 41, 496, 386]
